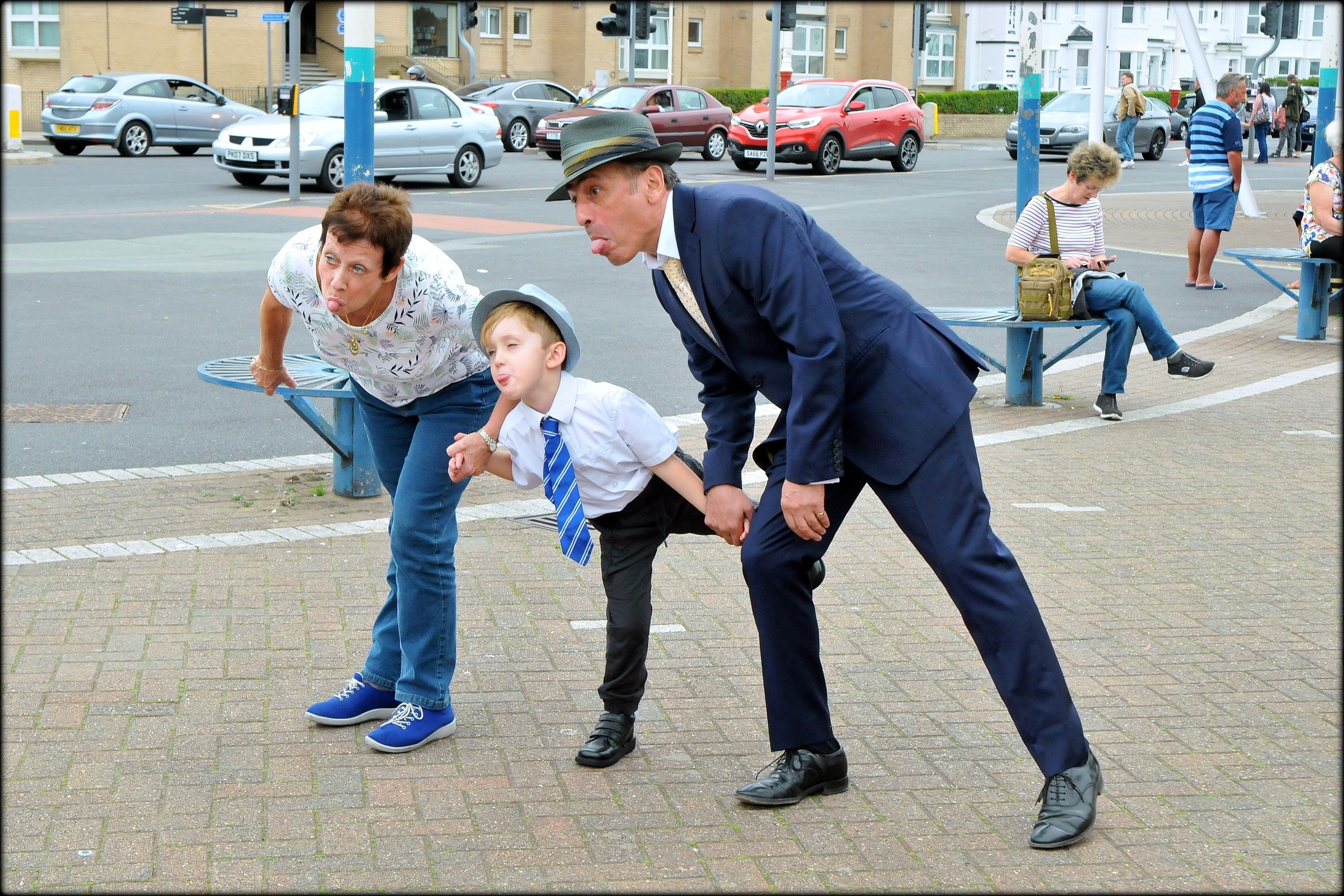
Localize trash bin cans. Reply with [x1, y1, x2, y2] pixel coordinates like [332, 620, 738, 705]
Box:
[2, 84, 23, 152]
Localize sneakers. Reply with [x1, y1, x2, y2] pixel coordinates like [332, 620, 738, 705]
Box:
[1121, 159, 1135, 169]
[365, 699, 455, 753]
[1179, 161, 1190, 167]
[1093, 391, 1122, 420]
[304, 672, 404, 725]
[1166, 353, 1216, 381]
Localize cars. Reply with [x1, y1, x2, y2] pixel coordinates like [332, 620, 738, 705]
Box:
[971, 81, 1019, 91]
[1175, 86, 1317, 139]
[1147, 96, 1189, 141]
[533, 82, 734, 163]
[1137, 85, 1166, 92]
[1294, 96, 1319, 152]
[464, 80, 578, 152]
[211, 73, 505, 193]
[39, 72, 266, 156]
[1005, 90, 1171, 161]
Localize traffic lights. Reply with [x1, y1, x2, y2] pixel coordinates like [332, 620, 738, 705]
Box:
[912, 3, 932, 52]
[462, 0, 479, 30]
[1259, 1, 1277, 37]
[635, 0, 657, 40]
[596, 1, 632, 38]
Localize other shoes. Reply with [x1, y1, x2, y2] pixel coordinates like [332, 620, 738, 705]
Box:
[1254, 161, 1268, 164]
[1285, 155, 1292, 158]
[1268, 153, 1280, 158]
[1294, 156, 1301, 159]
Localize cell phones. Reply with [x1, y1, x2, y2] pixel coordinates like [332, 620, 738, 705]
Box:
[1084, 260, 1114, 268]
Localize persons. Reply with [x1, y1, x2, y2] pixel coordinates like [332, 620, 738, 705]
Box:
[546, 112, 1105, 849]
[1286, 116, 1343, 291]
[407, 63, 429, 85]
[1178, 77, 1206, 166]
[447, 284, 826, 768]
[163, 82, 202, 102]
[1116, 72, 1146, 169]
[1005, 140, 1217, 419]
[578, 80, 667, 114]
[249, 184, 519, 754]
[1184, 72, 1247, 291]
[1235, 73, 1307, 164]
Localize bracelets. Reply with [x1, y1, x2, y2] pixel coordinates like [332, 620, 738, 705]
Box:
[251, 355, 285, 373]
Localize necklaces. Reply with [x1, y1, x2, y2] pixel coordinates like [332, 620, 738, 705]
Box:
[344, 287, 383, 336]
[342, 283, 385, 355]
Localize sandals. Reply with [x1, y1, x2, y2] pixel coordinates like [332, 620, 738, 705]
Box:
[1285, 283, 1300, 289]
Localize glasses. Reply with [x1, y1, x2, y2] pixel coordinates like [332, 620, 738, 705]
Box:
[1080, 179, 1108, 194]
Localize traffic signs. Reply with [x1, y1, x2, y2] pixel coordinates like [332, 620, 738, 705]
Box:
[206, 8, 238, 17]
[337, 23, 344, 34]
[336, 7, 345, 24]
[261, 14, 287, 22]
[172, 7, 204, 24]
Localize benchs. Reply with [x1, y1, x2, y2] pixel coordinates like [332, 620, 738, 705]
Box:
[1223, 248, 1343, 340]
[198, 354, 382, 500]
[926, 307, 1109, 407]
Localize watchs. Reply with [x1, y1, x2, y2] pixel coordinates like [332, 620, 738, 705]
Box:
[475, 427, 497, 455]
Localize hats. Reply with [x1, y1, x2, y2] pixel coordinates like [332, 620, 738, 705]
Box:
[470, 283, 580, 371]
[544, 111, 682, 202]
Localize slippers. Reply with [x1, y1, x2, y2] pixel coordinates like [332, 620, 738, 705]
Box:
[1185, 282, 1196, 288]
[1196, 278, 1227, 290]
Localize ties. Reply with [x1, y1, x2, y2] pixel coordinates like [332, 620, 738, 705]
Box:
[663, 258, 717, 348]
[540, 414, 594, 566]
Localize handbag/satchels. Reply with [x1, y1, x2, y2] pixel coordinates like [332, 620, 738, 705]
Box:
[1016, 254, 1072, 321]
[1252, 94, 1269, 124]
[1299, 106, 1310, 123]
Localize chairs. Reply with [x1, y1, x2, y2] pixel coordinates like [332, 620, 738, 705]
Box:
[379, 92, 406, 121]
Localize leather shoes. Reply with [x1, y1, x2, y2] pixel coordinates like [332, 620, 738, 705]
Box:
[574, 711, 635, 768]
[1029, 750, 1104, 848]
[735, 740, 848, 805]
[807, 560, 825, 590]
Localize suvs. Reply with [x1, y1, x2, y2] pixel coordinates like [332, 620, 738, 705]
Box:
[725, 77, 925, 175]
